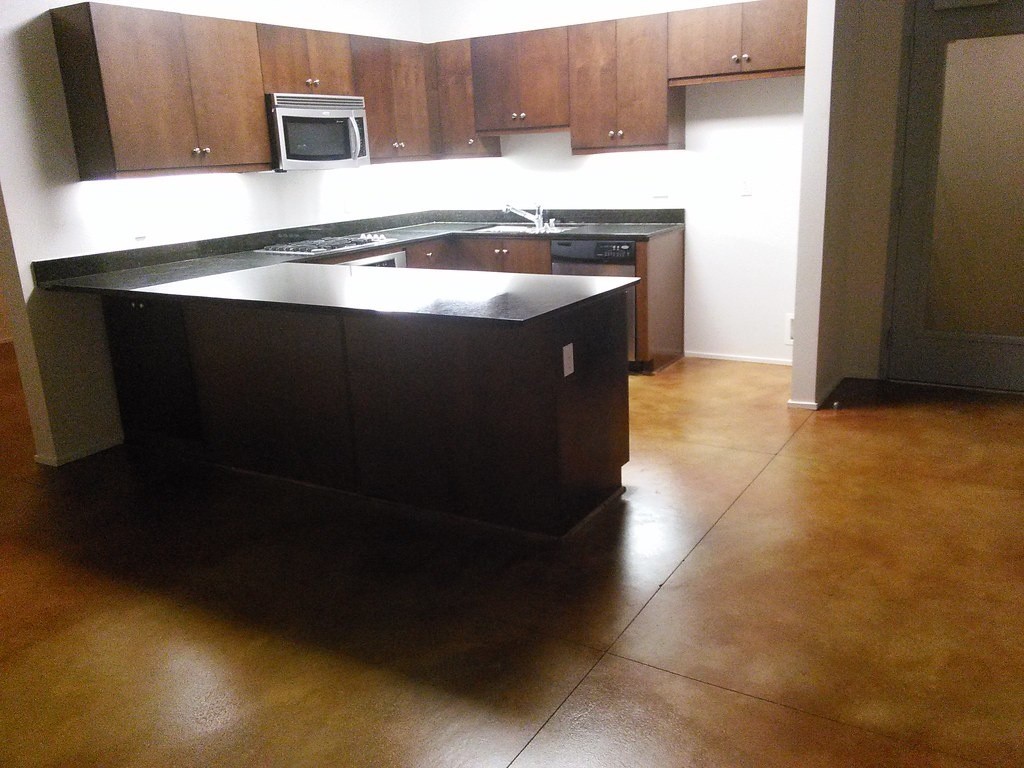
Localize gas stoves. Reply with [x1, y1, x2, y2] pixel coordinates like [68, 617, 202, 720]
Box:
[257, 233, 396, 257]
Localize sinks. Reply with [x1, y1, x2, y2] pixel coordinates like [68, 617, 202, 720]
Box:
[471, 225, 580, 234]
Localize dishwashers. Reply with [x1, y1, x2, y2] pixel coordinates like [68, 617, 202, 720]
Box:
[548, 240, 638, 360]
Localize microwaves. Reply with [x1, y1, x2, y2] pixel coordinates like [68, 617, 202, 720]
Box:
[267, 93, 370, 174]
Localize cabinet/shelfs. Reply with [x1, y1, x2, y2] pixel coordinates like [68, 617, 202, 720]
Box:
[102, 220, 684, 547]
[50, 0, 808, 180]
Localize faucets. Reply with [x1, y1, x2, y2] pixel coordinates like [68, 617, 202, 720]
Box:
[503, 203, 543, 228]
